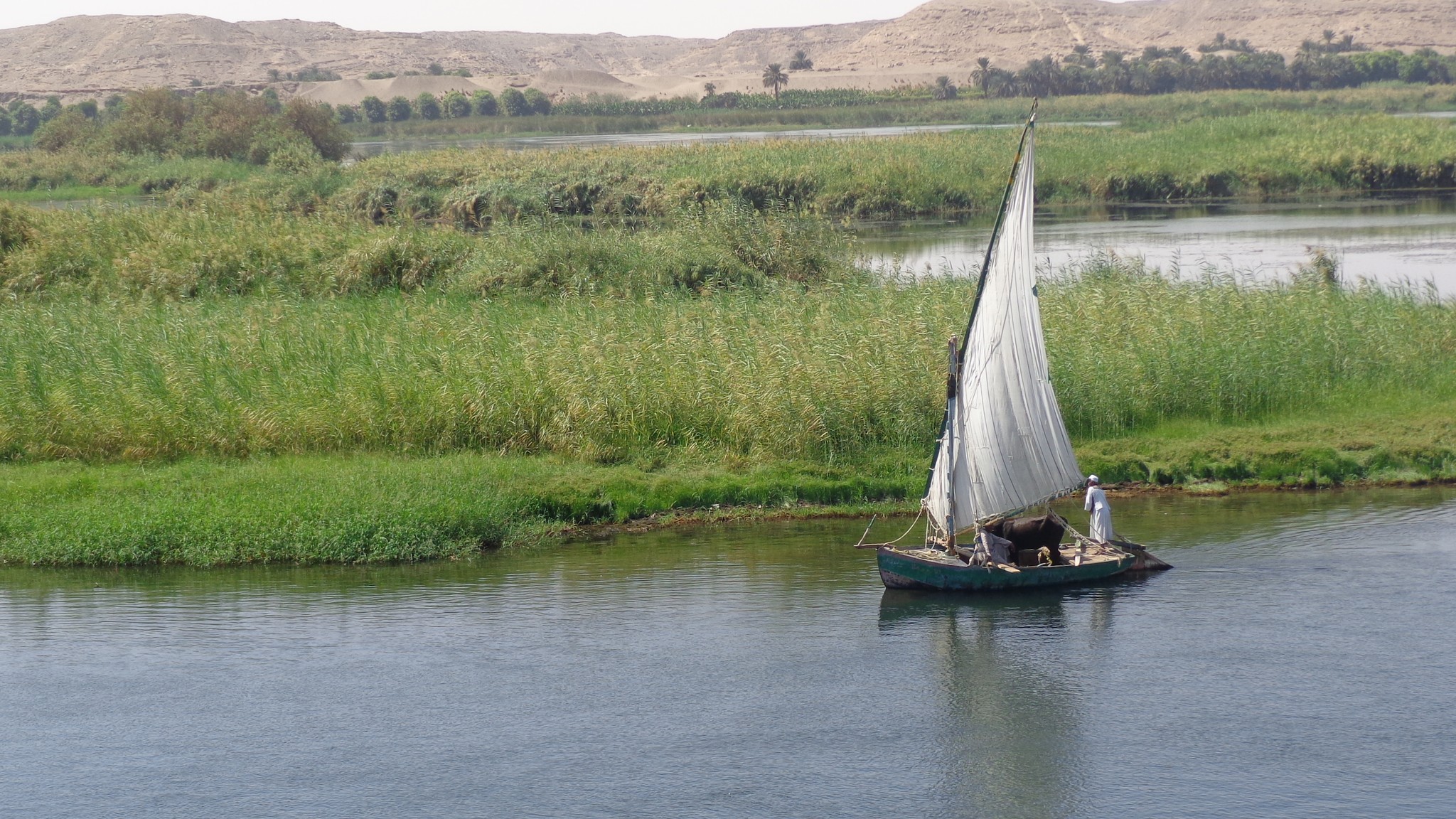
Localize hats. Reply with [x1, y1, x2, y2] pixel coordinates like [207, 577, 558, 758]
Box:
[1089, 475, 1098, 482]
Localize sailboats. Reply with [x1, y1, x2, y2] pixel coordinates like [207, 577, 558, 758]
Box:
[854, 97, 1175, 589]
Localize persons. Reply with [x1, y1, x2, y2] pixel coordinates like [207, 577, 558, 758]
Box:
[973, 519, 1014, 566]
[1084, 475, 1113, 555]
